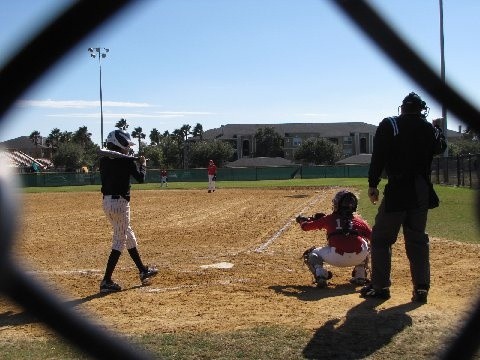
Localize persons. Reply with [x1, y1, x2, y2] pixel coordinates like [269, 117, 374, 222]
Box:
[300, 191, 373, 287]
[362, 93, 447, 306]
[207, 160, 217, 192]
[161, 169, 168, 188]
[98, 130, 159, 294]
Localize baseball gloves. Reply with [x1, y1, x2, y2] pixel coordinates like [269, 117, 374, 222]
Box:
[295, 212, 327, 223]
[212, 176, 217, 182]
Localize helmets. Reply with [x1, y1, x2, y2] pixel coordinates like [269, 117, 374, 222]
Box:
[106, 130, 135, 154]
[332, 191, 357, 214]
[398, 92, 430, 116]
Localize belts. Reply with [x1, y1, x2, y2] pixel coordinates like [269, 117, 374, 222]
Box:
[102, 194, 123, 200]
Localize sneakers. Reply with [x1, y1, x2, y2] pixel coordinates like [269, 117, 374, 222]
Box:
[412, 290, 428, 302]
[361, 287, 389, 298]
[317, 278, 327, 287]
[209, 190, 211, 193]
[100, 281, 121, 293]
[139, 269, 158, 281]
[209, 160, 213, 163]
[355, 279, 366, 284]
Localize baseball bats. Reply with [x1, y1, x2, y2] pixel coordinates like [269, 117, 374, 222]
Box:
[99, 147, 148, 164]
